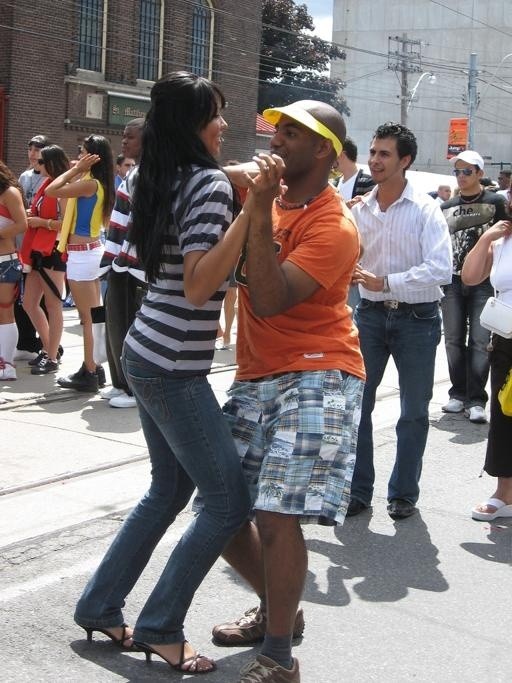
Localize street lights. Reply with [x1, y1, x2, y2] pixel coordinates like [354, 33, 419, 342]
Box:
[401, 72, 436, 126]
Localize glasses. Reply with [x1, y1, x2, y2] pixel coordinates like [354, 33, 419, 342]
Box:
[88, 134, 95, 142]
[451, 169, 473, 176]
[38, 159, 44, 166]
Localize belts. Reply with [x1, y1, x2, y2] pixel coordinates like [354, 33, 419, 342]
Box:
[383, 301, 401, 309]
[65, 242, 101, 251]
[0, 254, 18, 264]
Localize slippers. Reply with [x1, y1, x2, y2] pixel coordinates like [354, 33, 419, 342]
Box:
[471, 497, 512, 521]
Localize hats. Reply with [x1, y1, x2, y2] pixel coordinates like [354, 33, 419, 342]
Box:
[449, 151, 485, 170]
[27, 135, 49, 147]
[263, 105, 342, 156]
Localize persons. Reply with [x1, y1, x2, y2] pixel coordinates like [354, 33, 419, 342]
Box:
[212, 101, 366, 683]
[317, 122, 452, 527]
[432, 152, 512, 522]
[75, 71, 288, 676]
[1, 121, 375, 407]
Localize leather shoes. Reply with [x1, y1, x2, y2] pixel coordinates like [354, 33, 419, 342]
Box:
[345, 499, 364, 516]
[388, 500, 414, 517]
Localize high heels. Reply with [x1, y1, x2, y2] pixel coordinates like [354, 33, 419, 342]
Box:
[78, 622, 139, 650]
[132, 640, 217, 673]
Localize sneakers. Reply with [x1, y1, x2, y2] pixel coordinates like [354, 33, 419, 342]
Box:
[14, 346, 64, 374]
[58, 362, 99, 392]
[109, 393, 137, 408]
[442, 399, 464, 412]
[1, 358, 17, 381]
[235, 653, 300, 682]
[465, 406, 486, 423]
[212, 603, 304, 645]
[96, 366, 105, 384]
[101, 387, 125, 399]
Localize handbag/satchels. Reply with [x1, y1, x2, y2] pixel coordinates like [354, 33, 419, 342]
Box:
[498, 369, 512, 416]
[90, 307, 107, 365]
[479, 297, 511, 338]
